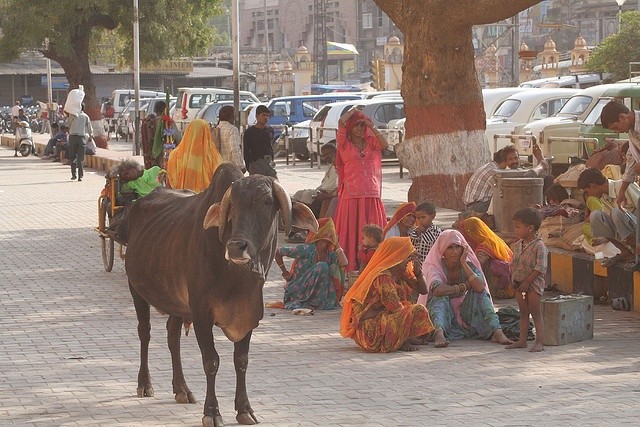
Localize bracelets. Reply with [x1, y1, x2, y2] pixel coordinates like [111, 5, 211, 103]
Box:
[415, 270, 424, 278]
[467, 273, 478, 283]
[279, 264, 284, 267]
[335, 247, 343, 254]
[371, 125, 376, 130]
[376, 132, 383, 137]
[460, 283, 467, 294]
[453, 285, 460, 294]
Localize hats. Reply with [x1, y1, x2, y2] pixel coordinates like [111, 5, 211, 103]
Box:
[256, 105, 270, 114]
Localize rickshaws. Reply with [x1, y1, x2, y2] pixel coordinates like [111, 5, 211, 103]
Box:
[94, 171, 166, 271]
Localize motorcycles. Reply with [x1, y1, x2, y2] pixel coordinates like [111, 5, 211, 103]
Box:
[0, 105, 13, 132]
[22, 105, 42, 131]
[14, 120, 32, 156]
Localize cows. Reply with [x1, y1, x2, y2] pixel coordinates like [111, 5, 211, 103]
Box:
[124, 161, 319, 426]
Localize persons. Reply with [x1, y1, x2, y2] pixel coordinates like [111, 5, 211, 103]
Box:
[408, 202, 443, 279]
[601, 100, 640, 272]
[528, 185, 583, 238]
[315, 149, 340, 219]
[12, 100, 22, 135]
[244, 105, 280, 182]
[340, 236, 435, 353]
[12, 109, 38, 157]
[380, 202, 417, 243]
[62, 102, 94, 181]
[357, 224, 383, 276]
[141, 100, 169, 166]
[109, 159, 166, 246]
[64, 133, 96, 164]
[504, 145, 520, 170]
[505, 208, 547, 352]
[461, 149, 507, 231]
[168, 118, 222, 193]
[275, 217, 348, 312]
[421, 228, 514, 348]
[215, 105, 246, 175]
[283, 142, 336, 238]
[576, 168, 640, 267]
[463, 216, 515, 299]
[102, 102, 114, 118]
[36, 100, 68, 162]
[580, 191, 609, 247]
[331, 106, 390, 271]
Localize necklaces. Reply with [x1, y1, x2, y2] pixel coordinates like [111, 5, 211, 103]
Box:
[353, 141, 366, 158]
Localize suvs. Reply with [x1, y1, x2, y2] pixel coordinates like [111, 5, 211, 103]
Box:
[307, 99, 373, 161]
[513, 83, 640, 162]
[197, 100, 256, 128]
[485, 87, 585, 162]
[348, 100, 406, 156]
[118, 98, 177, 133]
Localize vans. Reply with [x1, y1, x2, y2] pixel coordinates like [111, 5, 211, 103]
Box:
[112, 89, 173, 120]
[518, 73, 609, 86]
[174, 87, 263, 132]
[265, 94, 362, 141]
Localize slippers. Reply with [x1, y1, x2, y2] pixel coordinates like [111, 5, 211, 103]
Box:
[544, 283, 562, 291]
[623, 260, 640, 271]
[608, 297, 628, 311]
[601, 253, 627, 267]
